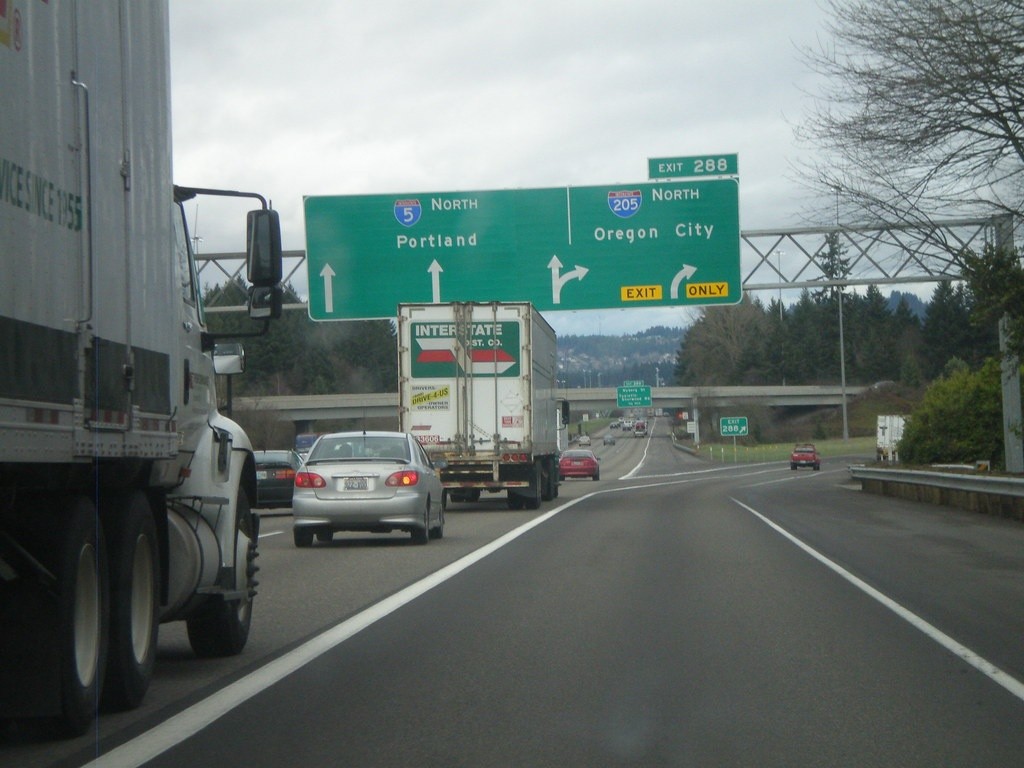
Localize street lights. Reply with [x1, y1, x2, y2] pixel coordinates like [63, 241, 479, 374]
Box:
[776, 247, 786, 323]
[655, 366, 660, 389]
[830, 183, 852, 442]
[556, 369, 603, 388]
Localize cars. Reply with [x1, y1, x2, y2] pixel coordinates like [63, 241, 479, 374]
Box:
[291, 432, 446, 545]
[609, 416, 624, 429]
[634, 430, 644, 438]
[253, 450, 304, 510]
[578, 436, 591, 447]
[603, 435, 616, 445]
[559, 450, 601, 481]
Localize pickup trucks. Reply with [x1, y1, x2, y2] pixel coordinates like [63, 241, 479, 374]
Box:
[788, 443, 821, 471]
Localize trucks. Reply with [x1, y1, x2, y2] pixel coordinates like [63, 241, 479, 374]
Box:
[294, 433, 317, 458]
[876, 415, 912, 458]
[396, 298, 570, 508]
[623, 420, 632, 430]
[0, 3, 281, 739]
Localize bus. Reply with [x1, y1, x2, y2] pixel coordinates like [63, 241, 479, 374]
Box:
[636, 421, 647, 435]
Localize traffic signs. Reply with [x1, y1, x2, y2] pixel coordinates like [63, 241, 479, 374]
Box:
[720, 418, 748, 435]
[303, 182, 741, 319]
